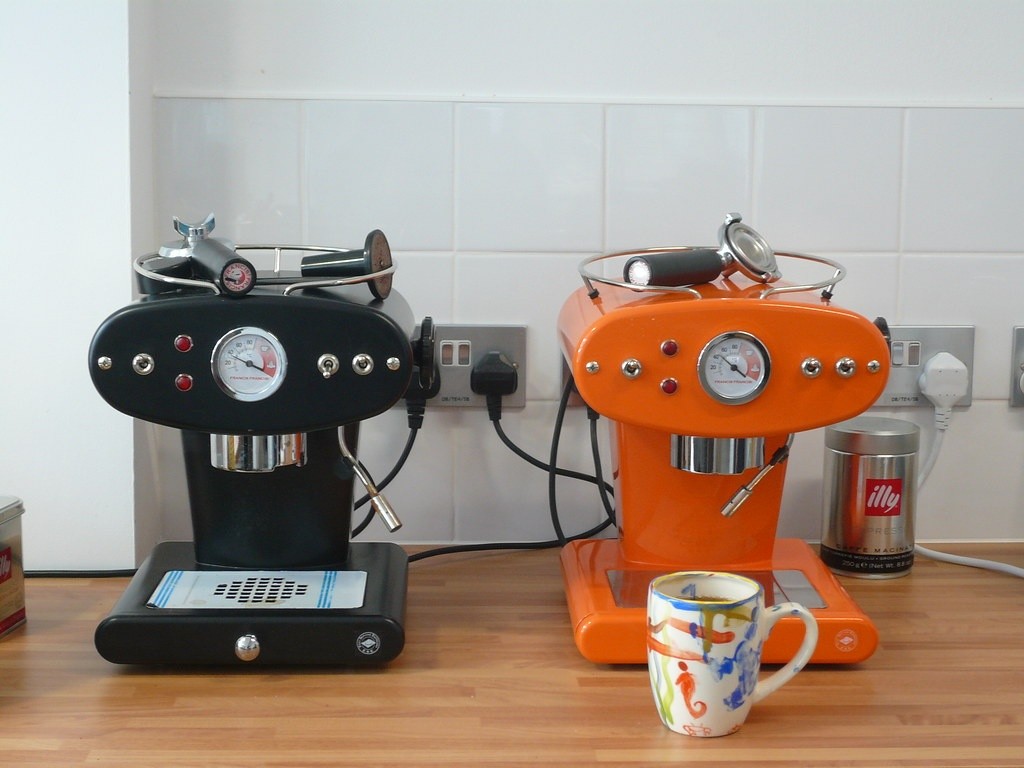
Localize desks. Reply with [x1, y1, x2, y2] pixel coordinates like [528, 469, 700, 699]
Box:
[0, 537, 1024, 768]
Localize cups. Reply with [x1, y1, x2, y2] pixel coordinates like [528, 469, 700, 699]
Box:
[646, 570, 820, 737]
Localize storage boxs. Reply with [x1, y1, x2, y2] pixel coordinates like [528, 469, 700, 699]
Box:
[0, 494, 28, 638]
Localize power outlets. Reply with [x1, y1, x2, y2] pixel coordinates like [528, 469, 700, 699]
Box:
[401, 322, 527, 410]
[868, 326, 975, 410]
